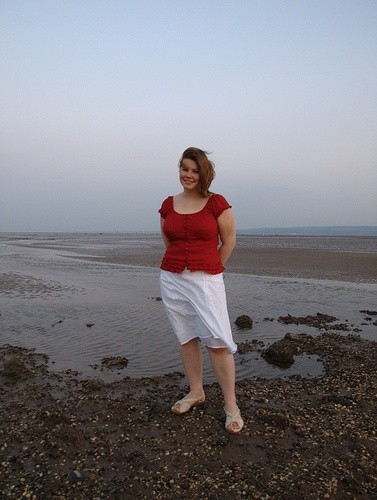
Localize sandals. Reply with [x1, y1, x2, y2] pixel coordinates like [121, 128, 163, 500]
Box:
[224, 404, 243, 433]
[170, 392, 206, 414]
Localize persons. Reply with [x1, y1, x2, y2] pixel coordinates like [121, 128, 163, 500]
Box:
[158, 148, 245, 435]
